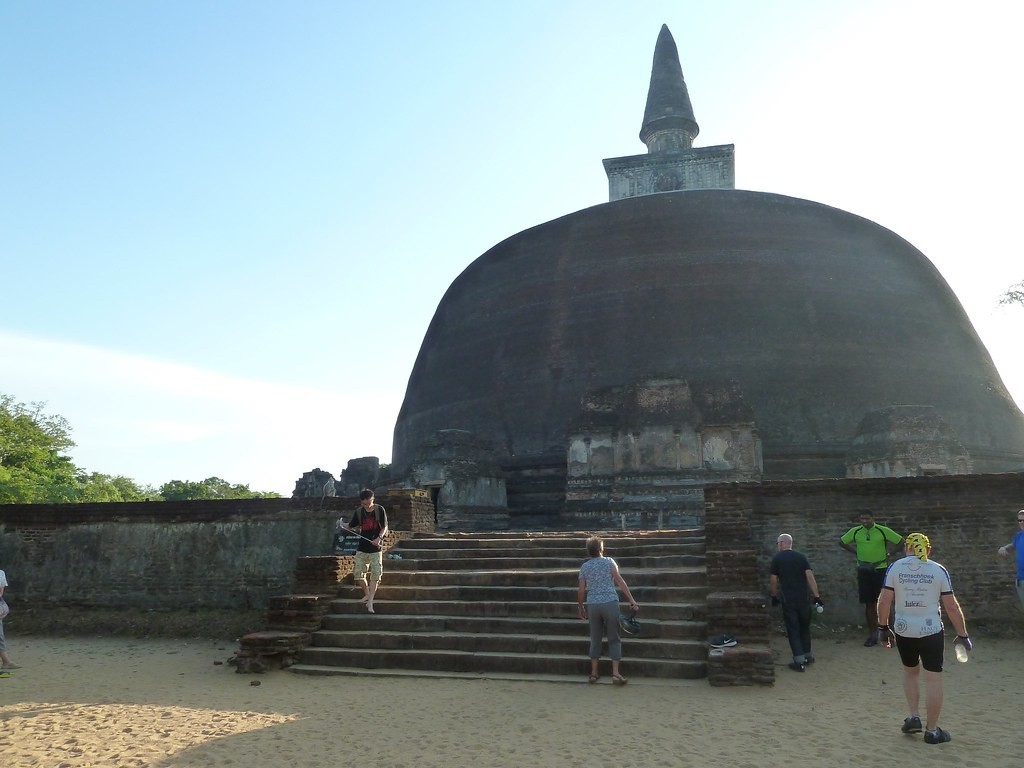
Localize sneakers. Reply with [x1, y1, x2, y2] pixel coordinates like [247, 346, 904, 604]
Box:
[901, 716, 922, 733]
[709, 633, 737, 647]
[924, 727, 951, 744]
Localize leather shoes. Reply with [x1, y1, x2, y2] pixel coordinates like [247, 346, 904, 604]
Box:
[789, 663, 805, 672]
[805, 656, 815, 664]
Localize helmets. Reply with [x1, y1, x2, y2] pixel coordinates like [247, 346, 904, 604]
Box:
[620, 610, 639, 635]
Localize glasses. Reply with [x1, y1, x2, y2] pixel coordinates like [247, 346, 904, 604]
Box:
[1017, 519, 1024, 522]
[776, 541, 784, 544]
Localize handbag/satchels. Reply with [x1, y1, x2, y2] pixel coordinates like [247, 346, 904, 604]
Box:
[856, 558, 887, 574]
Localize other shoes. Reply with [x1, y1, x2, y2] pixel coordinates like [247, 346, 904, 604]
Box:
[864, 637, 878, 647]
[366, 603, 375, 613]
[362, 596, 369, 603]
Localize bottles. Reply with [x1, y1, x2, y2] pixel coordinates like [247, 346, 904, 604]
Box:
[954, 643, 968, 663]
[817, 606, 823, 613]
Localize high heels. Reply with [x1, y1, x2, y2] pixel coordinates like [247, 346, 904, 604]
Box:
[590, 675, 599, 683]
[612, 674, 627, 685]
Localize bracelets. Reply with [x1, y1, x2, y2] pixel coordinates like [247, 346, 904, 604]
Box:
[378, 536, 382, 540]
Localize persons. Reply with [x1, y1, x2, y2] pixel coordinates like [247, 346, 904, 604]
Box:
[997, 510, 1024, 606]
[336, 491, 388, 613]
[838, 509, 906, 647]
[768, 533, 825, 672]
[877, 533, 972, 744]
[577, 536, 638, 684]
[0, 570, 22, 668]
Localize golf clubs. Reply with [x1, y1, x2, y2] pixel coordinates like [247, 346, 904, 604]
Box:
[335, 517, 383, 551]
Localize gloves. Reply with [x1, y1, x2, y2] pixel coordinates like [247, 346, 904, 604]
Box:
[771, 595, 780, 608]
[813, 598, 825, 608]
